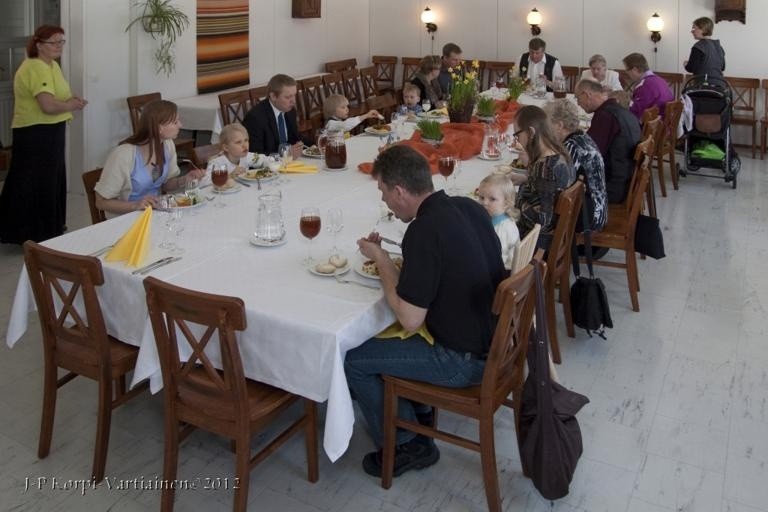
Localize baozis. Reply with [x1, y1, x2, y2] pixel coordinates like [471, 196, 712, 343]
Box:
[316, 263, 335, 274]
[328, 254, 347, 268]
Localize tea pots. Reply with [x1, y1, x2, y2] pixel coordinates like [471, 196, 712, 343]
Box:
[318, 120, 346, 169]
[253, 187, 287, 241]
[480, 126, 502, 160]
[552, 74, 567, 98]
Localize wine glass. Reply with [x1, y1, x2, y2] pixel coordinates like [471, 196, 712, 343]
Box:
[210, 163, 229, 208]
[153, 199, 184, 256]
[184, 177, 198, 218]
[267, 143, 291, 187]
[495, 75, 504, 94]
[437, 153, 462, 193]
[298, 206, 321, 265]
[421, 99, 431, 121]
[325, 206, 343, 258]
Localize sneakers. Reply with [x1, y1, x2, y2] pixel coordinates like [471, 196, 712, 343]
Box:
[362, 432, 440, 477]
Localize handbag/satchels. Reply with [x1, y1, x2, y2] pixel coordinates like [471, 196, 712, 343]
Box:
[516, 373, 589, 500]
[570, 277, 613, 330]
[634, 214, 665, 259]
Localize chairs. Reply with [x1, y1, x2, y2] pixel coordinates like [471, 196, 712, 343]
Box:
[81, 167, 107, 225]
[125, 91, 198, 165]
[510, 225, 557, 382]
[142, 277, 319, 512]
[322, 52, 767, 183]
[541, 173, 585, 363]
[21, 241, 151, 484]
[568, 101, 685, 311]
[216, 65, 397, 146]
[381, 248, 549, 510]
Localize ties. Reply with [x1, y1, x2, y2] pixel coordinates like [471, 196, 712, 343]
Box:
[278, 112, 286, 144]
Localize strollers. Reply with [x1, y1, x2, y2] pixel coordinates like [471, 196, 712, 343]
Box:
[674, 77, 742, 190]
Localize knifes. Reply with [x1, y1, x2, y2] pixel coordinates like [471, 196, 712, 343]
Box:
[132, 256, 183, 277]
[378, 234, 403, 249]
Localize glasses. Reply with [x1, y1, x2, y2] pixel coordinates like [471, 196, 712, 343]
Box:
[43, 40, 65, 45]
[513, 130, 522, 140]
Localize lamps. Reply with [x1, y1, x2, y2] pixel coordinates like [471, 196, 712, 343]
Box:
[525, 6, 540, 36]
[419, 6, 437, 35]
[646, 13, 662, 45]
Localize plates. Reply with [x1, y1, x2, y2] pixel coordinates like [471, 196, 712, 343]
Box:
[302, 148, 323, 159]
[354, 253, 404, 281]
[249, 239, 286, 247]
[212, 183, 240, 194]
[238, 170, 279, 181]
[161, 197, 205, 210]
[506, 144, 526, 155]
[307, 264, 351, 278]
[509, 165, 527, 173]
[362, 111, 439, 137]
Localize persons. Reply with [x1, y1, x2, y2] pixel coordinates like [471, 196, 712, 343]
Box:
[316, 95, 384, 143]
[242, 73, 314, 156]
[199, 121, 278, 188]
[683, 17, 726, 90]
[396, 33, 680, 205]
[0, 24, 89, 247]
[543, 97, 609, 234]
[510, 103, 575, 253]
[340, 144, 511, 480]
[479, 171, 521, 275]
[91, 99, 205, 219]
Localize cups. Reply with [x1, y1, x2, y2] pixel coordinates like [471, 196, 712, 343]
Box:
[522, 72, 547, 99]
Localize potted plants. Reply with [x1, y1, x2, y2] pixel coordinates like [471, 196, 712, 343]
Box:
[125, 0, 191, 77]
